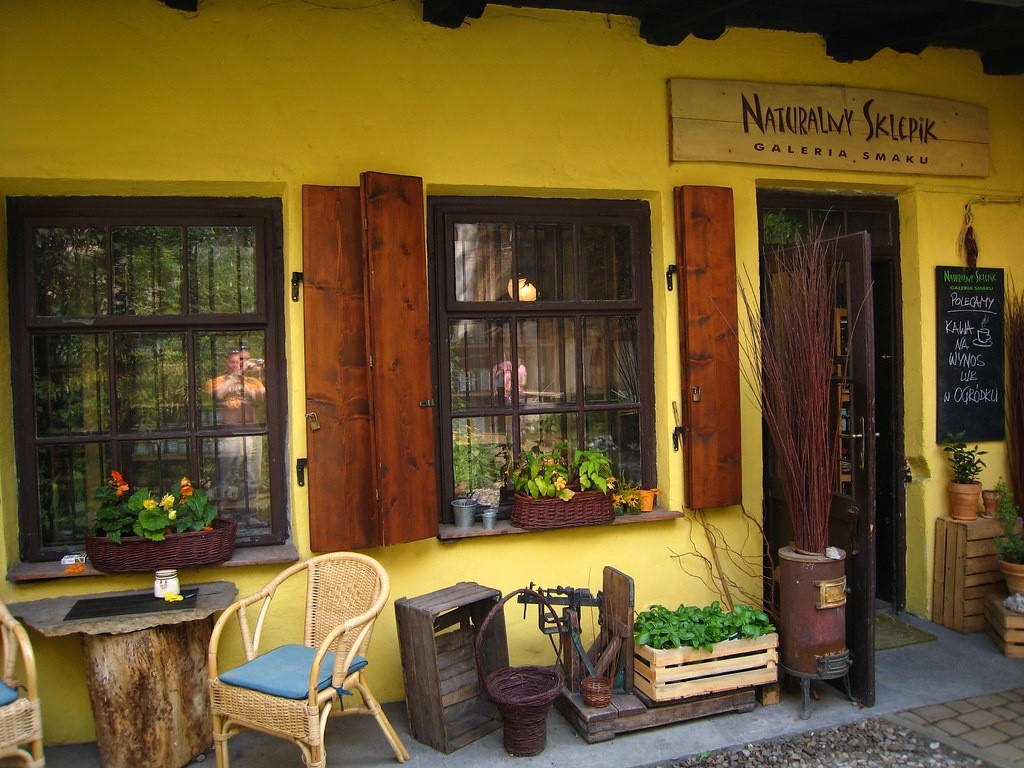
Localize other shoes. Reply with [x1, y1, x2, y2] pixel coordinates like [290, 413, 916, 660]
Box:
[247, 519, 270, 528]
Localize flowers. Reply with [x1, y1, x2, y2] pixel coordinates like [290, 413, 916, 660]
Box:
[612, 483, 641, 508]
[511, 442, 575, 503]
[94, 468, 219, 545]
[570, 445, 617, 497]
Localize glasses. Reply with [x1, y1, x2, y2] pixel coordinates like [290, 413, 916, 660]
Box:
[230, 348, 239, 353]
[241, 345, 249, 350]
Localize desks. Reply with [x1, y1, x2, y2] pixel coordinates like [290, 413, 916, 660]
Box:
[8, 578, 239, 767]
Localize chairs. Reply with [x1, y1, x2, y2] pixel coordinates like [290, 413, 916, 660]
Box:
[0, 597, 46, 768]
[208, 548, 411, 768]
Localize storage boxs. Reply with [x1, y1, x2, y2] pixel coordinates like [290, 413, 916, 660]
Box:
[395, 582, 509, 757]
[61, 555, 88, 564]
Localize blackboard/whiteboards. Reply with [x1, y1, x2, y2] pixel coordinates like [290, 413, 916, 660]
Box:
[933, 265, 1007, 445]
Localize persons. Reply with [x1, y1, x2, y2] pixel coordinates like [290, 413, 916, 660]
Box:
[206, 344, 269, 529]
[491, 354, 526, 408]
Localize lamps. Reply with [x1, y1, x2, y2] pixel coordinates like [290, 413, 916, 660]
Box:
[508, 273, 540, 303]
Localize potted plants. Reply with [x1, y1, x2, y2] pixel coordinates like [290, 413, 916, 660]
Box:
[633, 600, 778, 705]
[940, 429, 1024, 597]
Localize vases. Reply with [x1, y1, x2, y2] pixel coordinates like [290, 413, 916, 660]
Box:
[613, 501, 640, 517]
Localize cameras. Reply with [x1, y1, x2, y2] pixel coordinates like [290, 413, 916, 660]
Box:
[248, 359, 260, 370]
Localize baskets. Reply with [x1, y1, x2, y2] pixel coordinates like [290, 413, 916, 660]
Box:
[475, 589, 565, 757]
[84, 517, 237, 572]
[580, 675, 612, 708]
[510, 484, 616, 530]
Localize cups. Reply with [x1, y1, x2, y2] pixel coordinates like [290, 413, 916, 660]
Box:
[639, 489, 659, 512]
[483, 509, 498, 524]
[481, 514, 497, 530]
[154, 570, 180, 598]
